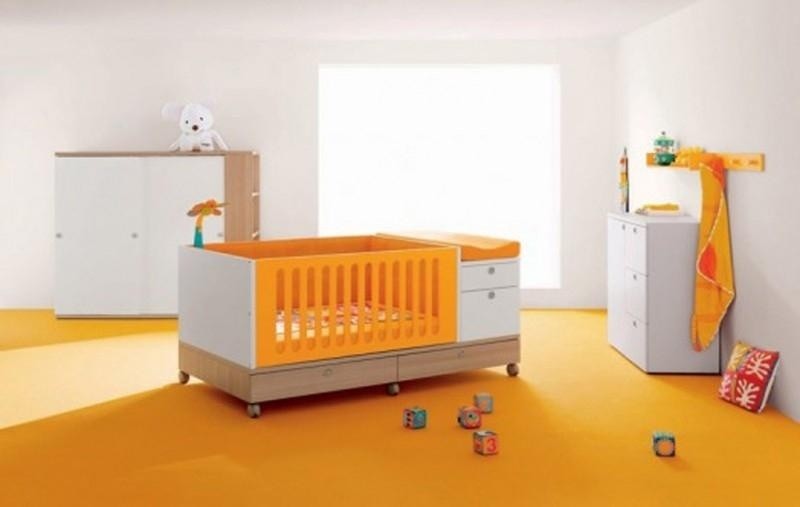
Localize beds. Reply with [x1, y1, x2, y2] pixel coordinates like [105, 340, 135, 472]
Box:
[178, 236, 520, 419]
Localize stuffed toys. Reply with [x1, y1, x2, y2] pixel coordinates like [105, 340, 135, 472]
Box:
[165, 102, 231, 153]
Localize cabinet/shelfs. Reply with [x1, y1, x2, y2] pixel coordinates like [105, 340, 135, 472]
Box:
[606, 214, 720, 376]
[53, 149, 260, 321]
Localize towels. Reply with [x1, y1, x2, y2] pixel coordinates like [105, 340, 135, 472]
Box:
[693, 162, 735, 351]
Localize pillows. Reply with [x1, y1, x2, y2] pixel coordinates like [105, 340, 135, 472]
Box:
[720, 341, 779, 414]
